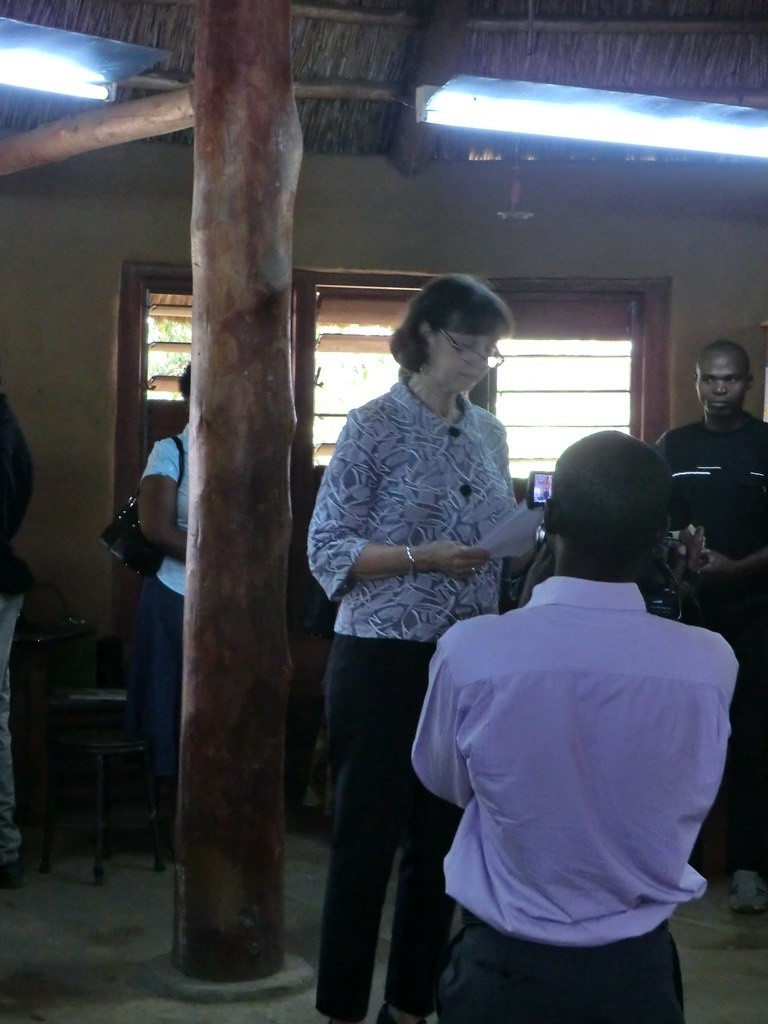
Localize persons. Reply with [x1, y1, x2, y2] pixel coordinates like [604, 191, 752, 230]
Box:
[136, 365, 192, 865]
[306, 272, 517, 1024]
[0, 374, 34, 889]
[413, 430, 739, 1024]
[652, 339, 768, 914]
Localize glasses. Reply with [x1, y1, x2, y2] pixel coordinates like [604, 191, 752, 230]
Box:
[438, 328, 504, 369]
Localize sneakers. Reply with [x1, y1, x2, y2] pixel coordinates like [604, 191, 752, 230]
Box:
[730, 869, 768, 914]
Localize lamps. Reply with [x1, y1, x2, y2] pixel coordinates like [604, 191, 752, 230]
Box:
[1, 17, 167, 104]
[415, 75, 765, 158]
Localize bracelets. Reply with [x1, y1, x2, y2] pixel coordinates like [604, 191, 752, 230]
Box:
[404, 545, 419, 582]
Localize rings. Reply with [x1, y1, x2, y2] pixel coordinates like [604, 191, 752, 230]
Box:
[472, 567, 475, 576]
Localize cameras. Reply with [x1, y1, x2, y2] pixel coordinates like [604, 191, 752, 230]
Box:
[526, 470, 554, 511]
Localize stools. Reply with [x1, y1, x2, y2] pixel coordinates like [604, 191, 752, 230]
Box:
[39, 726, 161, 888]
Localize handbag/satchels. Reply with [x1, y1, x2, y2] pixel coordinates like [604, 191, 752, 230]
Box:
[99, 433, 185, 579]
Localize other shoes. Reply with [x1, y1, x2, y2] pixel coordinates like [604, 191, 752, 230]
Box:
[376, 1003, 426, 1024]
[0, 859, 28, 890]
